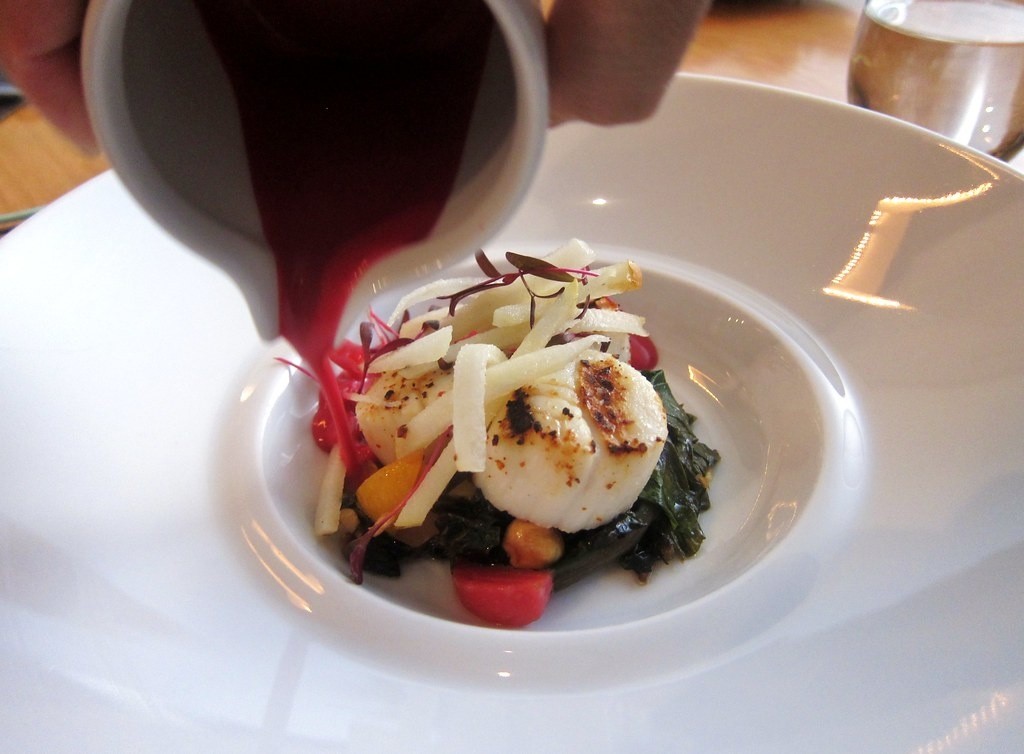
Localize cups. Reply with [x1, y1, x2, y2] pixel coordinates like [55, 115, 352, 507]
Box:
[846, 0, 1024, 164]
[80, 0, 553, 346]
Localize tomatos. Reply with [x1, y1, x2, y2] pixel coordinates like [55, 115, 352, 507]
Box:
[450, 565, 552, 626]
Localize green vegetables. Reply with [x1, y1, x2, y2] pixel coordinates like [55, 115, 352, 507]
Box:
[346, 367, 722, 590]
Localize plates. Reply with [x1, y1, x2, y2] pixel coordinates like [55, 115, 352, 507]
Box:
[1, 80, 1024, 754]
